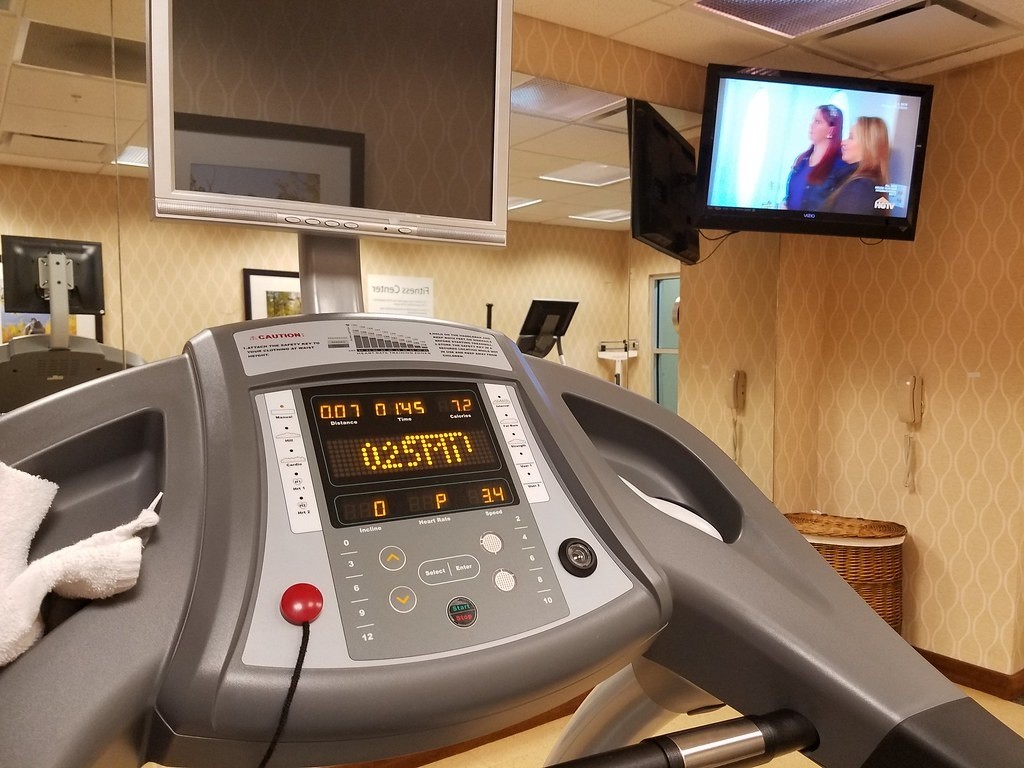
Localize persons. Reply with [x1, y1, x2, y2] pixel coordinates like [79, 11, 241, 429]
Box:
[817, 116, 894, 217]
[781, 104, 857, 212]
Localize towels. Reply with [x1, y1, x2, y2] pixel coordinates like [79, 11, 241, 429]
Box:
[0, 454, 169, 667]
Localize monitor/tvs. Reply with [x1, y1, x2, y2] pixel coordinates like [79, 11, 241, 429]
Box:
[519, 300, 579, 336]
[144, 0, 514, 245]
[625, 99, 700, 265]
[1, 234, 105, 316]
[692, 63, 934, 242]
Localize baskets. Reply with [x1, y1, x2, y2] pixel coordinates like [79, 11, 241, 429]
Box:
[784, 512, 908, 636]
